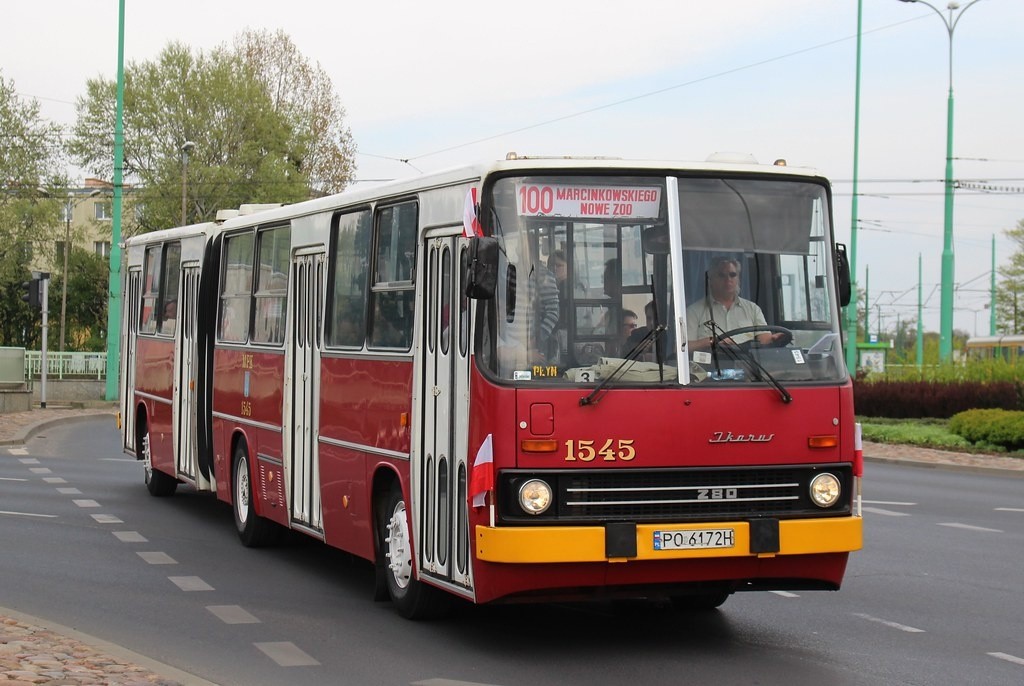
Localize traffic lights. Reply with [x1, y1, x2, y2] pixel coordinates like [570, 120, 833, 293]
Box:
[21, 279, 40, 308]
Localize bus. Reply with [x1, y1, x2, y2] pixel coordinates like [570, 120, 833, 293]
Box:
[114, 153, 864, 623]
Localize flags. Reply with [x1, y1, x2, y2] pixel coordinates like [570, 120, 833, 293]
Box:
[854, 423, 863, 476]
[463, 188, 484, 238]
[466, 434, 493, 511]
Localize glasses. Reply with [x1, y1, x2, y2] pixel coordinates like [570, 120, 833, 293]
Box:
[717, 273, 737, 277]
[624, 324, 638, 329]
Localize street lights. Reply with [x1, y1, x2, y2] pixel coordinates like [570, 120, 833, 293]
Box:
[35, 185, 100, 353]
[181, 141, 195, 226]
[897, 0, 990, 368]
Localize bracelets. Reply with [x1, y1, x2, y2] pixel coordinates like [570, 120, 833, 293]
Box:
[710, 337, 713, 345]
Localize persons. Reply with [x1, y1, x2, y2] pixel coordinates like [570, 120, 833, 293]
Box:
[486, 233, 617, 373]
[674, 256, 785, 353]
[622, 301, 658, 361]
